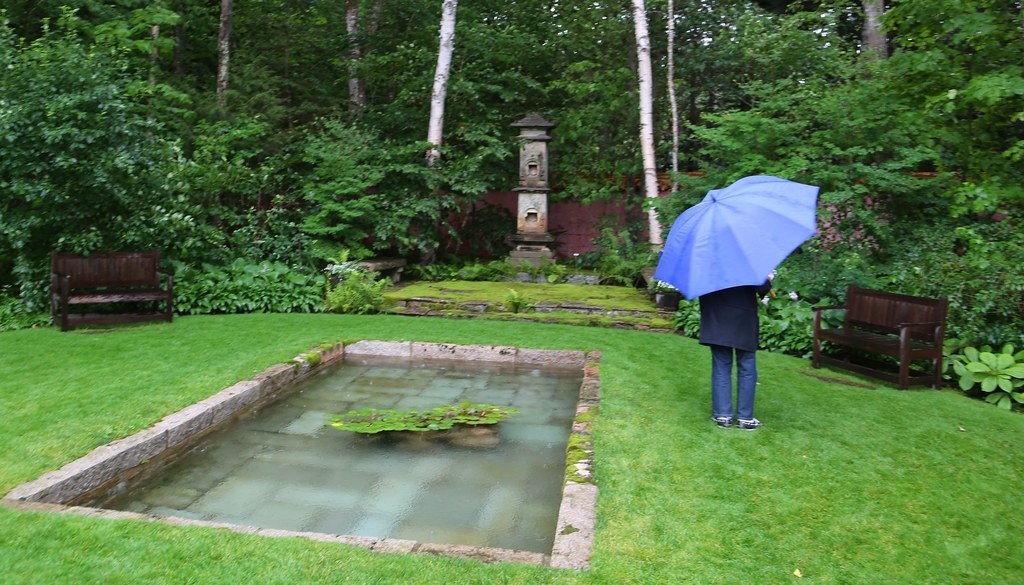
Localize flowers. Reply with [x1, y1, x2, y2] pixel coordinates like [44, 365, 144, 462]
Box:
[647, 276, 679, 294]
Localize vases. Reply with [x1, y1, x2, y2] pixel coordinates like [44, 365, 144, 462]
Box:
[656, 291, 680, 312]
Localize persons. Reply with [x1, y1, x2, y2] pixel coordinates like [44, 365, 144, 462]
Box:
[699, 272, 774, 432]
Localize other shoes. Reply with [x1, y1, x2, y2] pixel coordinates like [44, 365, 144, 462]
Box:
[711, 415, 734, 430]
[737, 417, 763, 431]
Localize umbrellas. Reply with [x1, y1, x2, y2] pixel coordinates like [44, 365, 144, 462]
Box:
[653, 175, 820, 301]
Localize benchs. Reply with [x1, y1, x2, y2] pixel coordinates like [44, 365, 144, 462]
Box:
[342, 256, 408, 285]
[51, 247, 174, 332]
[641, 266, 657, 287]
[810, 285, 949, 391]
